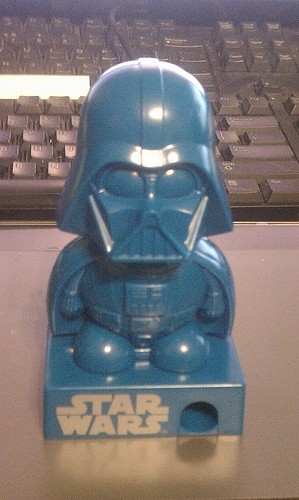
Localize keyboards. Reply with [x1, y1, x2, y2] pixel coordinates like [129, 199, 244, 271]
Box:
[0, 79, 299, 222]
[0, 17, 299, 99]
